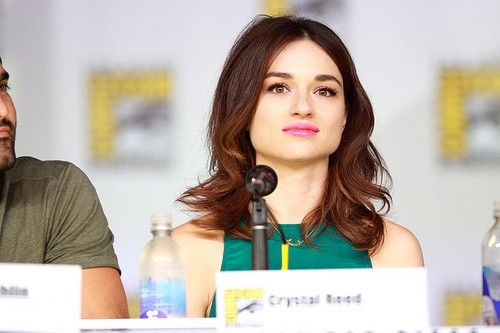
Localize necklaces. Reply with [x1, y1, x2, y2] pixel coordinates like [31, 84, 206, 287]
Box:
[262, 219, 323, 246]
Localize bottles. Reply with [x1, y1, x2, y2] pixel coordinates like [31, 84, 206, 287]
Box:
[482, 199, 500, 325]
[139, 210, 190, 318]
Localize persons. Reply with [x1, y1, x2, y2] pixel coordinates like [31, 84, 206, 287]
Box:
[143, 10, 424, 317]
[0, 57, 131, 319]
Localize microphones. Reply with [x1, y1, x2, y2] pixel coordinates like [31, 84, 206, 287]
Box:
[245, 165, 277, 270]
